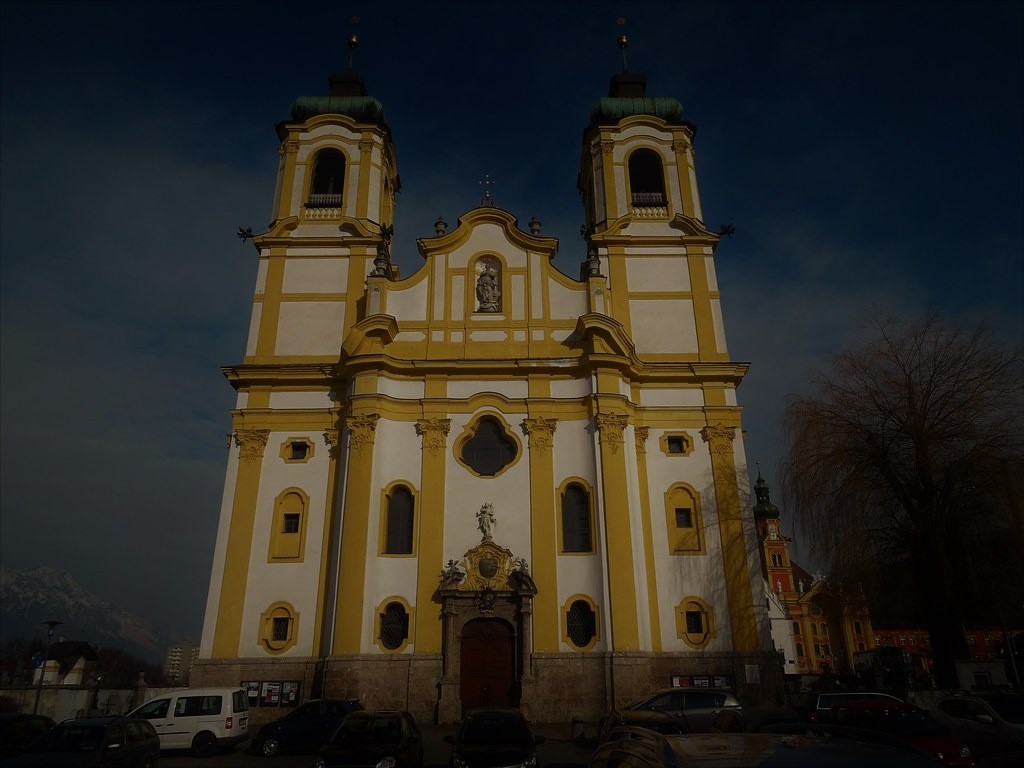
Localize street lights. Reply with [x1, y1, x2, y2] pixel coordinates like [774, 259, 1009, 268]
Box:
[32, 621, 61, 715]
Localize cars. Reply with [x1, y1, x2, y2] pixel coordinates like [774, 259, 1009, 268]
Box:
[589, 725, 942, 768]
[444, 705, 546, 768]
[251, 698, 366, 757]
[604, 686, 746, 733]
[313, 710, 425, 768]
[0, 713, 55, 760]
[753, 688, 1024, 768]
[0, 717, 160, 768]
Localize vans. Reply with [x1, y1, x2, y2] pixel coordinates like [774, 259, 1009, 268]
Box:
[122, 687, 250, 758]
